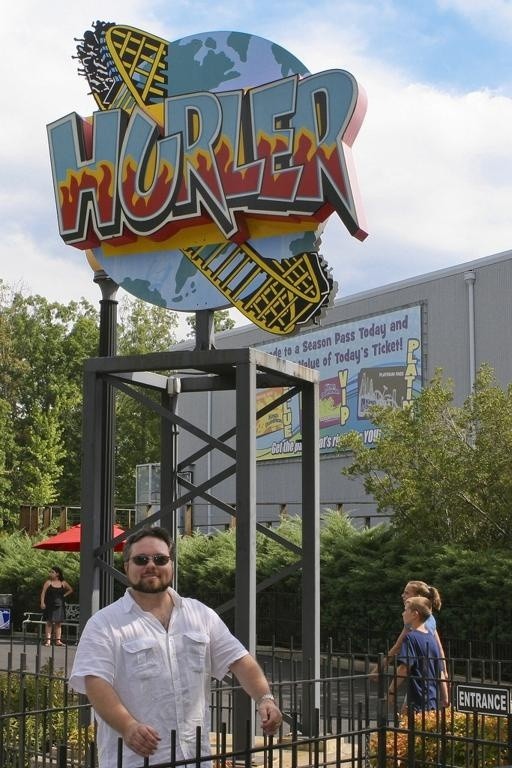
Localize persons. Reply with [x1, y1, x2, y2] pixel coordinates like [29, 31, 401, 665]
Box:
[40, 566, 75, 647]
[367, 580, 450, 714]
[387, 596, 450, 719]
[66, 526, 285, 768]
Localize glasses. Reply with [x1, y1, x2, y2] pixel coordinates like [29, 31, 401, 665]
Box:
[128, 554, 172, 566]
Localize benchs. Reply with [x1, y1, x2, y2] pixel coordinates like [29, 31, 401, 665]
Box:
[21, 602, 80, 645]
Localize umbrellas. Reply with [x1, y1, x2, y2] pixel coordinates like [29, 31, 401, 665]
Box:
[29, 520, 136, 554]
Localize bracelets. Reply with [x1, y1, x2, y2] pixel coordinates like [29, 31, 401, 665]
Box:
[255, 693, 276, 706]
[388, 691, 395, 696]
